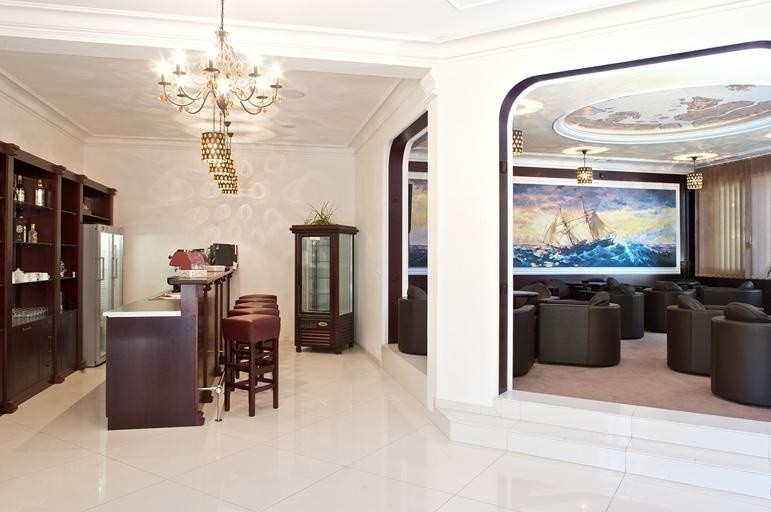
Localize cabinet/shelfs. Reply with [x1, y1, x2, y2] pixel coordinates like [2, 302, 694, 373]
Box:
[2, 141, 118, 423]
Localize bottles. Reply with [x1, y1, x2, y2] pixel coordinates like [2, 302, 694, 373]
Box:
[13, 175, 55, 244]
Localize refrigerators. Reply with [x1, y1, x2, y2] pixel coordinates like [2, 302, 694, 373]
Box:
[81, 226, 125, 366]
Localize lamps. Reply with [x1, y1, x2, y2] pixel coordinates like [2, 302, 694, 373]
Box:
[152, 0, 285, 195]
[687, 154, 703, 190]
[576, 148, 594, 184]
[512, 128, 523, 155]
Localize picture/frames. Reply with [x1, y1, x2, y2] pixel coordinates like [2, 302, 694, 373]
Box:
[407, 170, 681, 275]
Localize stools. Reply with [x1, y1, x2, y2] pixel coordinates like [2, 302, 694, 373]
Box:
[223, 294, 282, 417]
